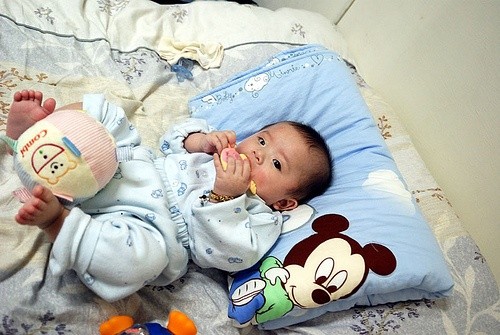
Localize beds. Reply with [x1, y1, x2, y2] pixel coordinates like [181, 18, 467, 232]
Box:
[0, 1, 499, 334]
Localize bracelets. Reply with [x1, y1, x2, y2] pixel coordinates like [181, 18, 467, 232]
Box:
[210, 190, 234, 202]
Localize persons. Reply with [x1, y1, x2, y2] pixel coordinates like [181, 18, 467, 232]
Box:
[6, 89, 334, 303]
